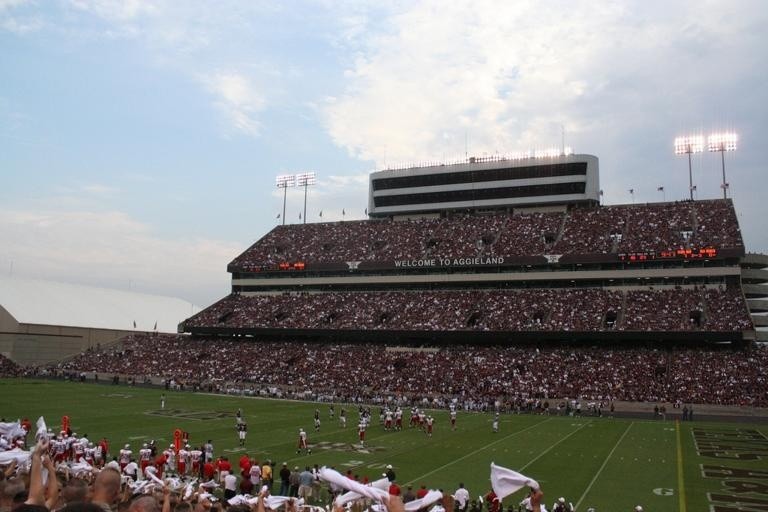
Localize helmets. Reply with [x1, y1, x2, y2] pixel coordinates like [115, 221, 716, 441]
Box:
[299, 419, 369, 432]
[371, 406, 504, 420]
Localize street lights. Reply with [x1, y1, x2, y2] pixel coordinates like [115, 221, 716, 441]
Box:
[297, 171, 318, 224]
[275, 174, 297, 225]
[674, 133, 705, 201]
[706, 130, 738, 199]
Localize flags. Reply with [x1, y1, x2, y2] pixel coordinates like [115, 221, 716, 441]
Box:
[688, 185, 696, 190]
[656, 186, 664, 191]
[134, 320, 157, 330]
[596, 189, 604, 197]
[720, 184, 729, 189]
[627, 188, 633, 194]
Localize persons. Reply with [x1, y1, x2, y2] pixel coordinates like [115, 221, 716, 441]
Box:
[1, 332, 185, 408]
[0, 408, 574, 511]
[180, 197, 766, 417]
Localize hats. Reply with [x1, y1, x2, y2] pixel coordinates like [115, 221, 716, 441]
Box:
[386, 464, 393, 469]
[557, 497, 566, 503]
[46, 427, 191, 452]
